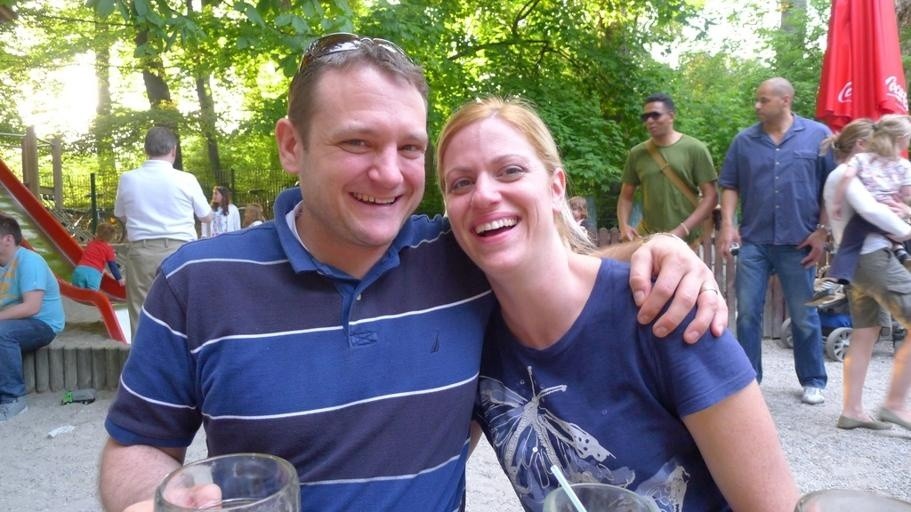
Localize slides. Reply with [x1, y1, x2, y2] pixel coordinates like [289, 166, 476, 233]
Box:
[0, 160, 132, 341]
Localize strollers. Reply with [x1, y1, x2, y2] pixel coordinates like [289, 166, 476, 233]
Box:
[776, 242, 906, 360]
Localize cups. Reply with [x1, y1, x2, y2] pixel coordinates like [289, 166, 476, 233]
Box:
[155, 452, 302, 512]
[797, 487, 910, 512]
[544, 483, 661, 512]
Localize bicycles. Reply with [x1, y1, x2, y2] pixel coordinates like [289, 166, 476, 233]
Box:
[51, 192, 123, 243]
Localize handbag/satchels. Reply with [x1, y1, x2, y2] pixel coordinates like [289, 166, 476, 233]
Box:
[699, 197, 721, 244]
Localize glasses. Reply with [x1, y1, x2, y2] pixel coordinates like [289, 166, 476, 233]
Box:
[294, 33, 415, 80]
[641, 112, 660, 122]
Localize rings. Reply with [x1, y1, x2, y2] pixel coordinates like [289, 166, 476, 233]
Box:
[700, 288, 719, 295]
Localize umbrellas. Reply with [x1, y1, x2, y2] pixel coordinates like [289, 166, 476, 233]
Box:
[818, 0, 909, 160]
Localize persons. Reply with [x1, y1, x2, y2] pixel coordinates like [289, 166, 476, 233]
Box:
[71, 223, 126, 291]
[716, 74, 841, 406]
[616, 93, 719, 256]
[243, 202, 266, 230]
[567, 195, 591, 255]
[434, 93, 802, 511]
[200, 186, 241, 241]
[97, 33, 731, 512]
[820, 119, 911, 434]
[112, 127, 216, 347]
[829, 111, 911, 269]
[1, 215, 66, 422]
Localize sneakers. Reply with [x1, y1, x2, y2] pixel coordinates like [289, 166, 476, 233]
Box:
[0, 396, 28, 423]
[805, 281, 835, 306]
[895, 248, 910, 271]
[878, 407, 910, 431]
[837, 415, 892, 429]
[802, 386, 824, 404]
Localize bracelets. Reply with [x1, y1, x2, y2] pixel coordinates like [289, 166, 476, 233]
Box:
[817, 223, 831, 236]
[679, 223, 691, 237]
